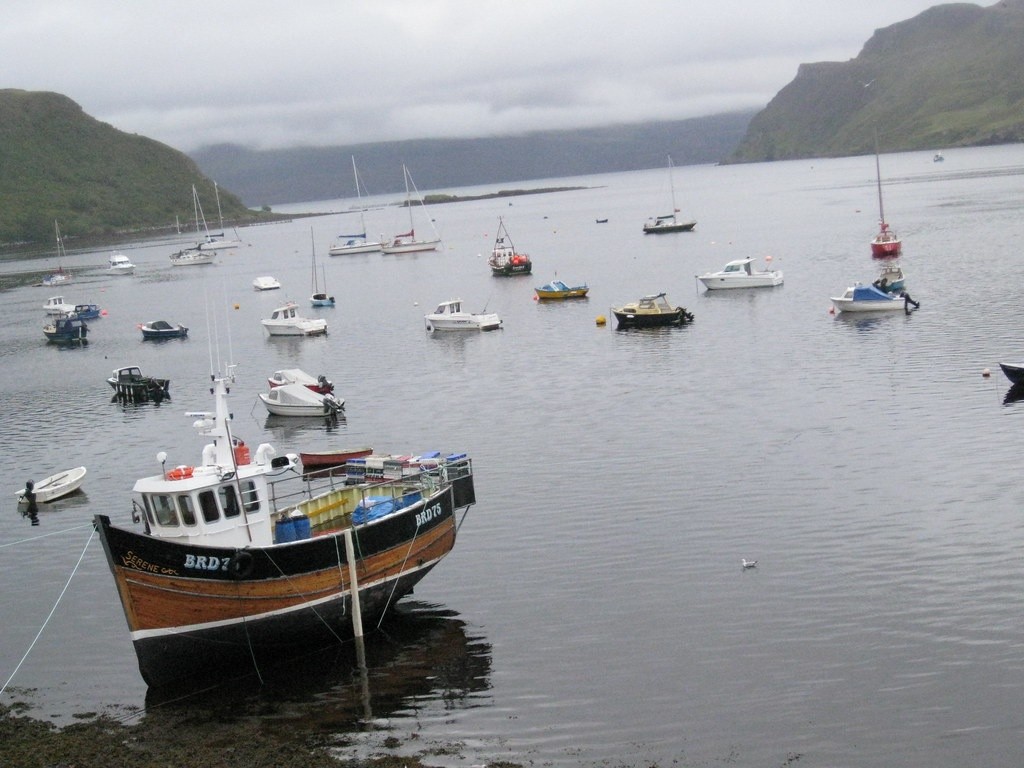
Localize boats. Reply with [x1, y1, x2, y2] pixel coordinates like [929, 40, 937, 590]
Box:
[14, 466, 88, 511]
[42, 296, 74, 313]
[70, 303, 99, 319]
[258, 383, 348, 417]
[43, 315, 87, 341]
[260, 304, 327, 336]
[138, 320, 188, 340]
[93, 360, 477, 689]
[486, 214, 531, 276]
[831, 264, 919, 316]
[697, 257, 783, 289]
[105, 367, 169, 394]
[254, 276, 281, 288]
[423, 297, 502, 331]
[106, 254, 136, 276]
[534, 279, 692, 328]
[267, 369, 334, 392]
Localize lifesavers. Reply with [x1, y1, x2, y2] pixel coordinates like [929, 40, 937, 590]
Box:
[229, 551, 254, 577]
[167, 466, 193, 481]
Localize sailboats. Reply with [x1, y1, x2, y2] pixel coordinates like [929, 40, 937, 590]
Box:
[866, 143, 902, 255]
[40, 152, 443, 307]
[647, 152, 694, 234]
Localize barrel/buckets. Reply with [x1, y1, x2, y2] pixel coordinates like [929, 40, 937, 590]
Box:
[291, 515, 311, 540]
[275, 520, 297, 543]
[233, 439, 250, 465]
[401, 488, 422, 508]
